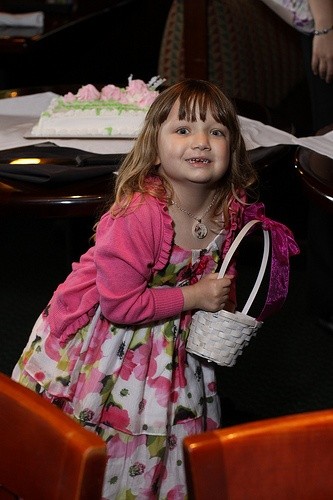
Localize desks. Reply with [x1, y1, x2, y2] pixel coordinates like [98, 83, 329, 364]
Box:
[0, 83, 295, 260]
[294, 124, 333, 332]
[0, 0, 130, 87]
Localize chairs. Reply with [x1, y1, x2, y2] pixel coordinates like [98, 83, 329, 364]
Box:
[183, 409, 333, 500]
[0, 372, 107, 500]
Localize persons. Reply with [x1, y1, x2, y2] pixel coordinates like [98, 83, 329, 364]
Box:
[10, 77, 260, 500]
[260, 0, 333, 136]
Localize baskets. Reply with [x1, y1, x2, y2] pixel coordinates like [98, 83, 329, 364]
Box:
[185, 220, 271, 367]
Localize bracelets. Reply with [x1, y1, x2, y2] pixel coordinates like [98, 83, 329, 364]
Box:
[313, 25, 333, 35]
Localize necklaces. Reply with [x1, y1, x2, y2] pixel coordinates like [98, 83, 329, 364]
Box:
[170, 192, 218, 240]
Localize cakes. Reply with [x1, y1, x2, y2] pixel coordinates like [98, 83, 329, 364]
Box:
[31, 75, 165, 135]
